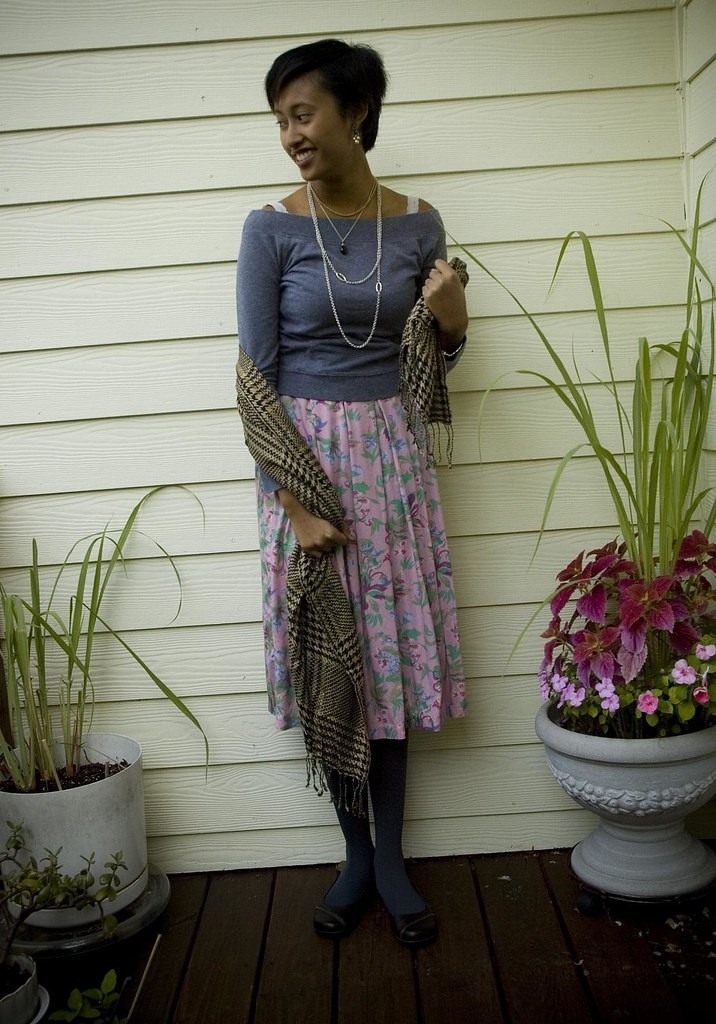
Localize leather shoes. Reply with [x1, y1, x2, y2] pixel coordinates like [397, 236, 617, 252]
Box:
[371, 854, 438, 946]
[313, 870, 374, 940]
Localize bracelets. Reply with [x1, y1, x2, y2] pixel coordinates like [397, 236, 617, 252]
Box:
[444, 335, 466, 361]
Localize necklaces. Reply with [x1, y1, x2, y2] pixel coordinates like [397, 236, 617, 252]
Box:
[308, 179, 382, 352]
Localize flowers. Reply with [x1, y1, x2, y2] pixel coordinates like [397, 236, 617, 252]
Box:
[429, 164, 716, 739]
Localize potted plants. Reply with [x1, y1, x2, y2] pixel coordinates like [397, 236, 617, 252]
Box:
[0, 820, 128, 1024]
[0, 484, 209, 929]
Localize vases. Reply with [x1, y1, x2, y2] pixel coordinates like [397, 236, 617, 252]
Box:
[534, 701, 716, 899]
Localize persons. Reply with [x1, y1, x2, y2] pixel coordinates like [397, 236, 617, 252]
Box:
[237, 38, 471, 953]
